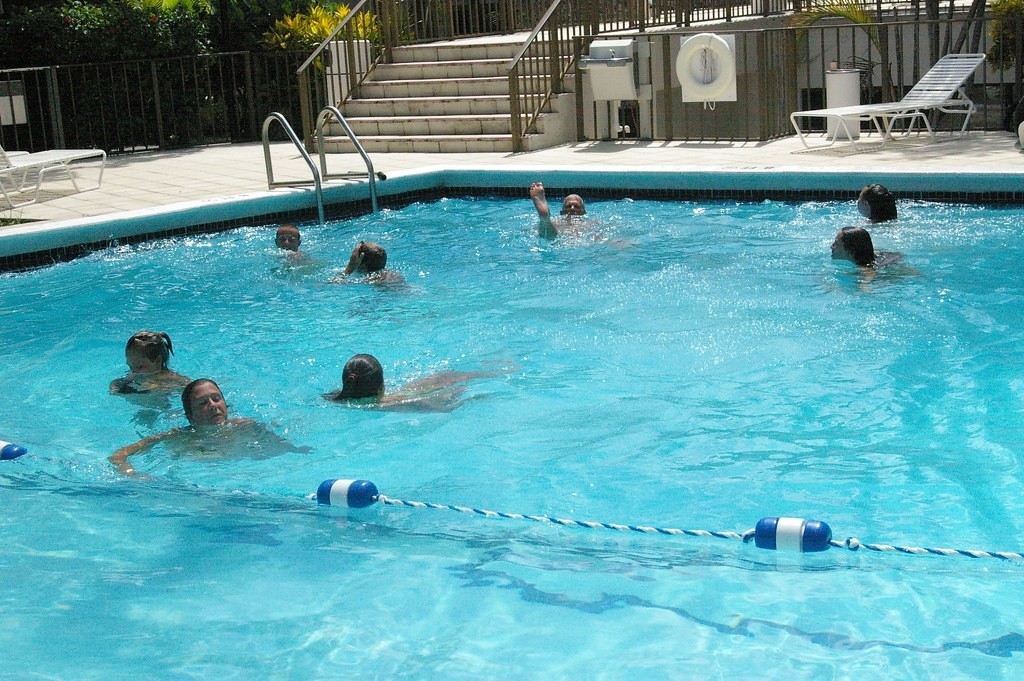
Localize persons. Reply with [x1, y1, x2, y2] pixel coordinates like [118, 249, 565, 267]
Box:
[831, 227, 877, 267]
[343, 239, 385, 277]
[181, 379, 229, 425]
[123, 331, 175, 376]
[532, 180, 586, 240]
[335, 354, 385, 400]
[855, 183, 899, 221]
[276, 225, 301, 250]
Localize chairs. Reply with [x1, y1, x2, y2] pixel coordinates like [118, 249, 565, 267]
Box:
[790, 53, 986, 153]
[0, 151, 30, 194]
[0, 145, 107, 208]
